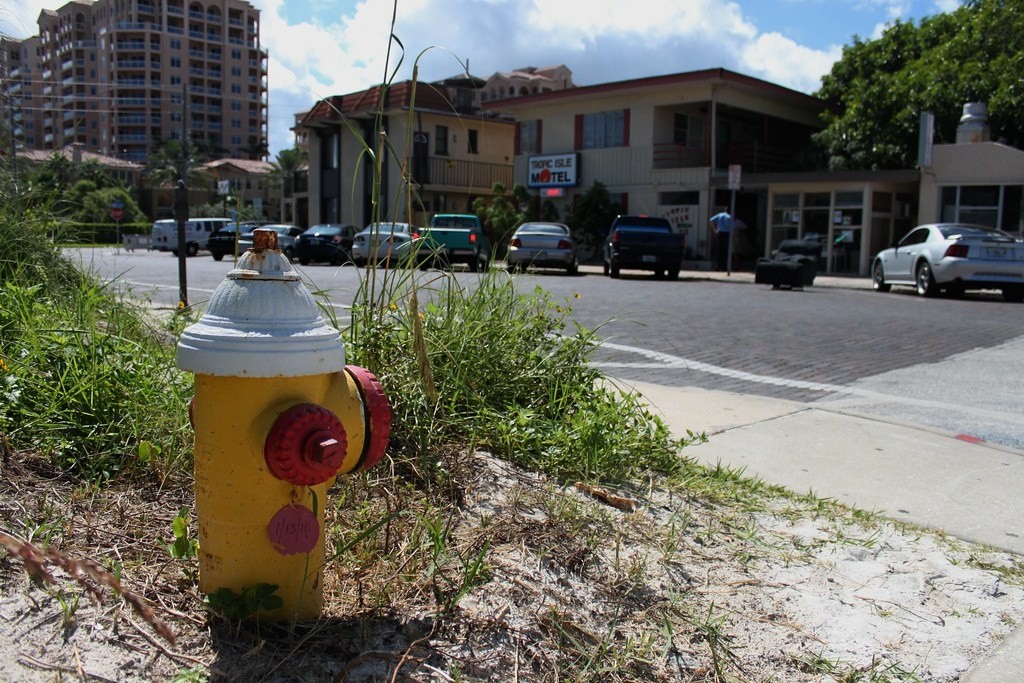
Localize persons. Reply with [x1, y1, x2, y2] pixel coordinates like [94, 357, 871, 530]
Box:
[709, 207, 737, 270]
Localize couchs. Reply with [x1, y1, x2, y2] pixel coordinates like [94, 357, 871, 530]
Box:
[753, 239, 823, 288]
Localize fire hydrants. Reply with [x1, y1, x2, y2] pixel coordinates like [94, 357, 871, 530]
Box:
[173, 225, 393, 633]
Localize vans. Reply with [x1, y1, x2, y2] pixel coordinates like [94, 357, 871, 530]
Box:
[151, 218, 233, 260]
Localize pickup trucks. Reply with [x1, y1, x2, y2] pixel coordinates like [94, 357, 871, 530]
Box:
[604, 215, 685, 281]
[415, 214, 491, 273]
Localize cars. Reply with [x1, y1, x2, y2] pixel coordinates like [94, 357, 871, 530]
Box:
[294, 224, 357, 266]
[238, 224, 302, 263]
[205, 222, 267, 263]
[352, 222, 419, 269]
[870, 224, 1024, 302]
[507, 222, 578, 276]
